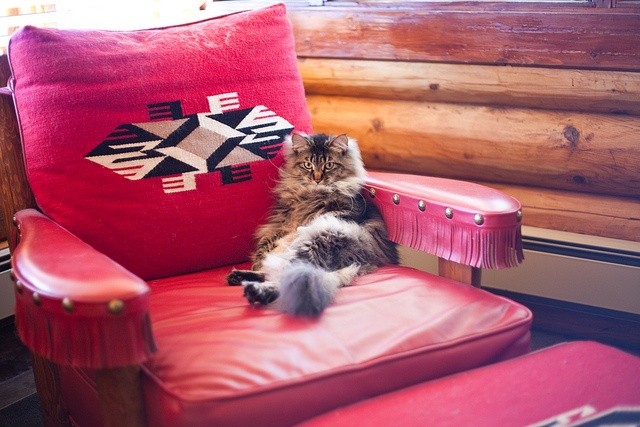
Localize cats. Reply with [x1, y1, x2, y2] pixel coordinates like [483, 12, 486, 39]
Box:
[225, 132, 400, 318]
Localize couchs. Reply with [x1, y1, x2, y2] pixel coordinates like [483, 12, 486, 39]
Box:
[295, 341, 640, 426]
[7, 3, 535, 426]
